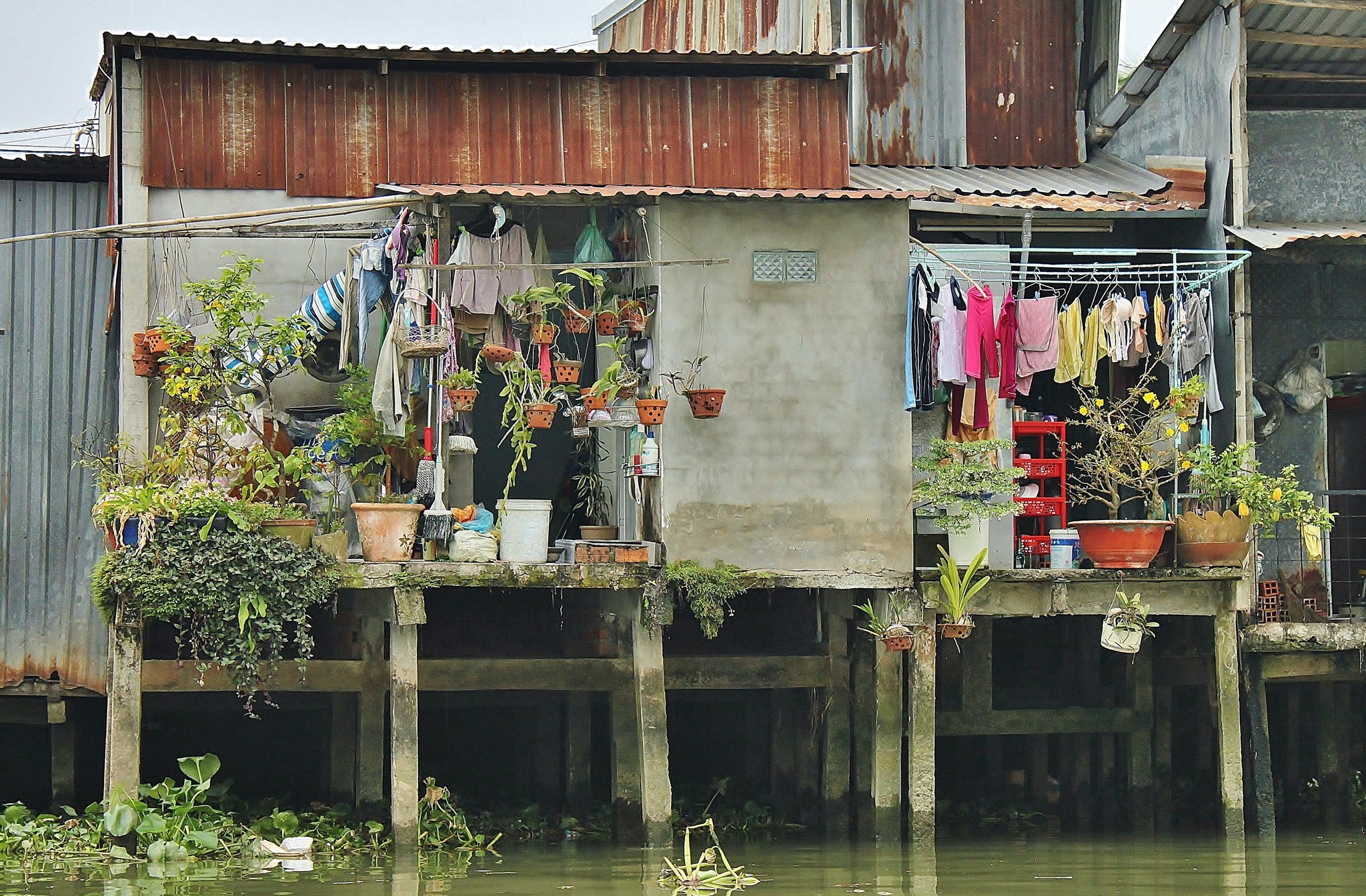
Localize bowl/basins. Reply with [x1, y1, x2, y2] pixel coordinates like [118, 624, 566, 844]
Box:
[547, 546, 566, 562]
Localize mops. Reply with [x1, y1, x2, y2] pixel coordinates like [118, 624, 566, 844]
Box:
[413, 239, 438, 501]
[1189, 394, 1214, 494]
[422, 379, 454, 544]
[448, 410, 479, 455]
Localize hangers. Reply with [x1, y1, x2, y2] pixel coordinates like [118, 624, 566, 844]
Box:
[911, 249, 1216, 315]
[370, 202, 515, 241]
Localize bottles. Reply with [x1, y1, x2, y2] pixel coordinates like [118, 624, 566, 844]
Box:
[629, 424, 659, 475]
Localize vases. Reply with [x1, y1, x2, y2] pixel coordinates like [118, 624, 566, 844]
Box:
[158, 364, 184, 384]
[132, 352, 156, 377]
[146, 328, 173, 356]
[132, 331, 151, 355]
[177, 333, 196, 357]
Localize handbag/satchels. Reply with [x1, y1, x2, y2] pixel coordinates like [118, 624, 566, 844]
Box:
[573, 205, 613, 265]
[1273, 343, 1334, 414]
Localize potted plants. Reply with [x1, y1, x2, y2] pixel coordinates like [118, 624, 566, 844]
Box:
[928, 541, 995, 643]
[1047, 318, 1341, 573]
[1100, 596, 1162, 655]
[68, 221, 728, 574]
[910, 431, 1030, 568]
[850, 588, 917, 653]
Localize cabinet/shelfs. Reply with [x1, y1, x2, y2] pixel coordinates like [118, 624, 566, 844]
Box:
[1013, 420, 1068, 557]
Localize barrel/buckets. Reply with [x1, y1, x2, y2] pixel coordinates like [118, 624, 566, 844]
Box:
[1049, 529, 1081, 569]
[1258, 556, 1263, 582]
[495, 499, 553, 563]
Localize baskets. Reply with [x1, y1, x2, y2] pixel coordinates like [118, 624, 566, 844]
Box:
[184, 296, 211, 327]
[390, 288, 451, 359]
